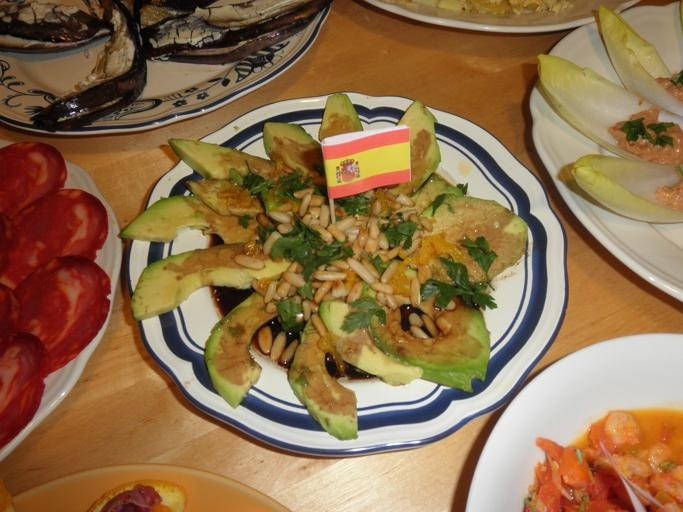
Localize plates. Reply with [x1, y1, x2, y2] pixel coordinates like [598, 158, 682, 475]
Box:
[128, 90, 570, 453]
[0, 2, 332, 133]
[531, 1, 682, 304]
[1, 141, 125, 461]
[468, 333, 681, 511]
[1, 463, 289, 510]
[369, 0, 640, 32]
